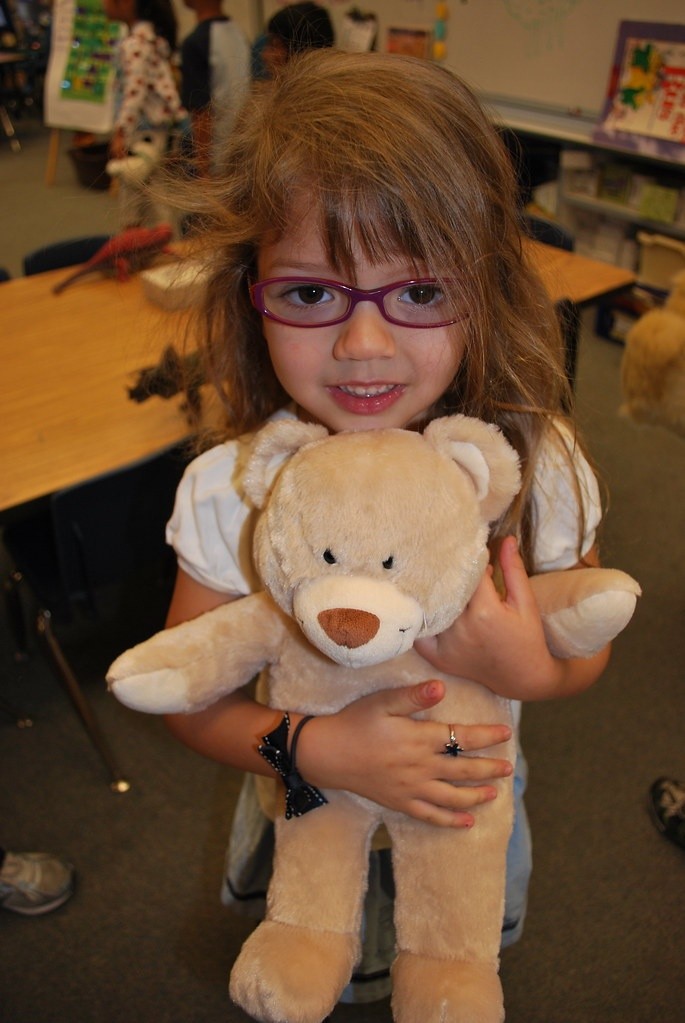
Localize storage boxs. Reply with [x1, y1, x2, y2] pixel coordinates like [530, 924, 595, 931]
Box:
[635, 230, 685, 291]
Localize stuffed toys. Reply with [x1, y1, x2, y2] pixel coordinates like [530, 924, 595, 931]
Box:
[104, 406, 645, 1023]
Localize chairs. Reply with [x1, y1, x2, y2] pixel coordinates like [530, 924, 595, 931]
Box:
[23, 235, 110, 276]
[515, 213, 577, 253]
[1, 457, 177, 793]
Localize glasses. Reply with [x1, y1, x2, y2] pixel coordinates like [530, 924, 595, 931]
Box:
[245, 275, 472, 329]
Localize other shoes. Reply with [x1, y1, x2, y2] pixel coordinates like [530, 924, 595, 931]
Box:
[644, 774, 685, 847]
[0, 850, 78, 916]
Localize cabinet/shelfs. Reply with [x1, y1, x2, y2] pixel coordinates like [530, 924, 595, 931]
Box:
[557, 141, 685, 299]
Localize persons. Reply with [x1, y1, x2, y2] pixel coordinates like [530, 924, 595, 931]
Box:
[163, 46, 612, 1023]
[66, 1, 337, 255]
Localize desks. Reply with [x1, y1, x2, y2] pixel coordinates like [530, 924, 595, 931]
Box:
[478, 96, 685, 166]
[0, 232, 637, 528]
[0, 52, 27, 152]
